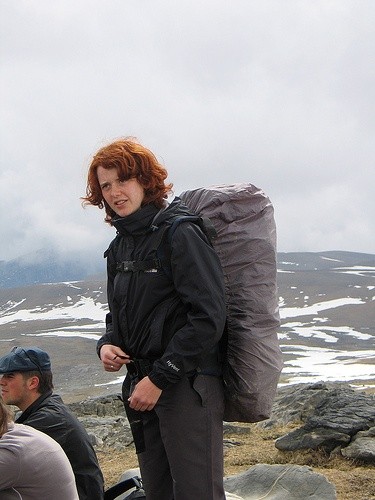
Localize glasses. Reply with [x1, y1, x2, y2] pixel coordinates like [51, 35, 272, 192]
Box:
[11, 346, 43, 374]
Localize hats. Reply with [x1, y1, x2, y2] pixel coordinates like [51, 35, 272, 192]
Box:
[0, 347, 51, 373]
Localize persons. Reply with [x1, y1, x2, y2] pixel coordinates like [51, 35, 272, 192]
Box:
[0, 394, 79, 500]
[80, 135, 227, 500]
[0, 345, 105, 500]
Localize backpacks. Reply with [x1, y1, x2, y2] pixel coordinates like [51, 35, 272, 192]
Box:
[111, 182, 282, 423]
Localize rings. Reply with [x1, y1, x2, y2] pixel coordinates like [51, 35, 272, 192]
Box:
[113, 355, 118, 360]
[111, 365, 114, 369]
